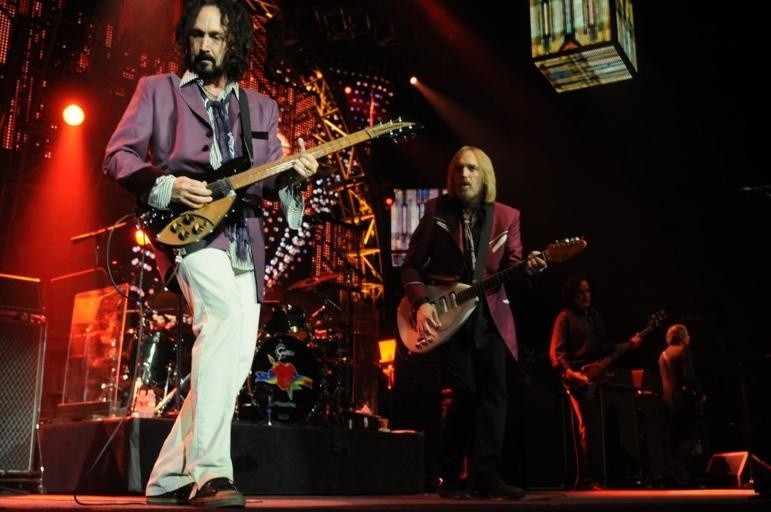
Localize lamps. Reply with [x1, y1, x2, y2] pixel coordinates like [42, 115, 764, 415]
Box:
[529, 0, 637, 93]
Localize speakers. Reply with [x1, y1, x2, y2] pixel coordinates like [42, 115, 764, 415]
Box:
[0, 273, 49, 485]
[704, 450, 771, 489]
[563, 380, 645, 481]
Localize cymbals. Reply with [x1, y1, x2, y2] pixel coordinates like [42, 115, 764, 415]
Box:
[287, 274, 339, 292]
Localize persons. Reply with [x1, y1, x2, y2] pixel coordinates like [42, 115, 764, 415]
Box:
[102, 0, 320, 507]
[659, 324, 707, 489]
[399, 146, 548, 499]
[547, 277, 642, 490]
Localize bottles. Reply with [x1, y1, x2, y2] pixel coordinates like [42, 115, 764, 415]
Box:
[135, 388, 157, 418]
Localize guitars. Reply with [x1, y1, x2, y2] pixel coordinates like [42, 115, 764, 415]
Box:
[397, 237, 586, 355]
[575, 309, 667, 401]
[134, 116, 418, 246]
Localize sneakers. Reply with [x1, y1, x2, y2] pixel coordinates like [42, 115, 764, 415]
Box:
[436, 481, 528, 502]
[145, 482, 195, 505]
[188, 477, 246, 508]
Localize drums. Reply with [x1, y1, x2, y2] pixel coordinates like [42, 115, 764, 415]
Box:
[241, 335, 322, 423]
[264, 302, 311, 340]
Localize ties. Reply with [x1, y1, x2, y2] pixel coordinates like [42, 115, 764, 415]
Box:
[461, 208, 478, 279]
[194, 83, 251, 260]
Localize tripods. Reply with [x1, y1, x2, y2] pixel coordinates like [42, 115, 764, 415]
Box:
[302, 350, 345, 422]
